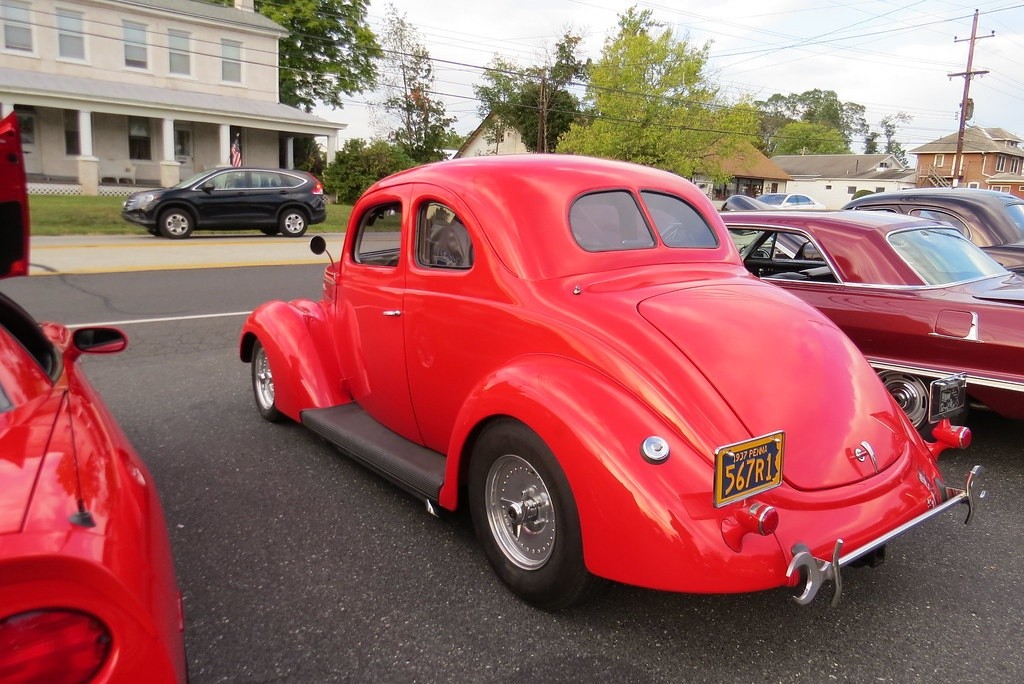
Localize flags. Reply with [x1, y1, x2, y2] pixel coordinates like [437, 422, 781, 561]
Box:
[230, 138, 242, 168]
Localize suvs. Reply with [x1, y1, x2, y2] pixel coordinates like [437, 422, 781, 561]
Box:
[119, 165, 328, 239]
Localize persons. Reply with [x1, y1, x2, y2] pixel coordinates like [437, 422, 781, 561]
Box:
[429, 213, 472, 268]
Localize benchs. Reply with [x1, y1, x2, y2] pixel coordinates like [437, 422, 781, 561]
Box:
[97, 159, 137, 185]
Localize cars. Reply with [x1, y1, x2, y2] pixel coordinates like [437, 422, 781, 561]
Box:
[757, 192, 829, 213]
[0, 98, 200, 684]
[709, 207, 1024, 435]
[719, 180, 1024, 287]
[235, 153, 992, 620]
